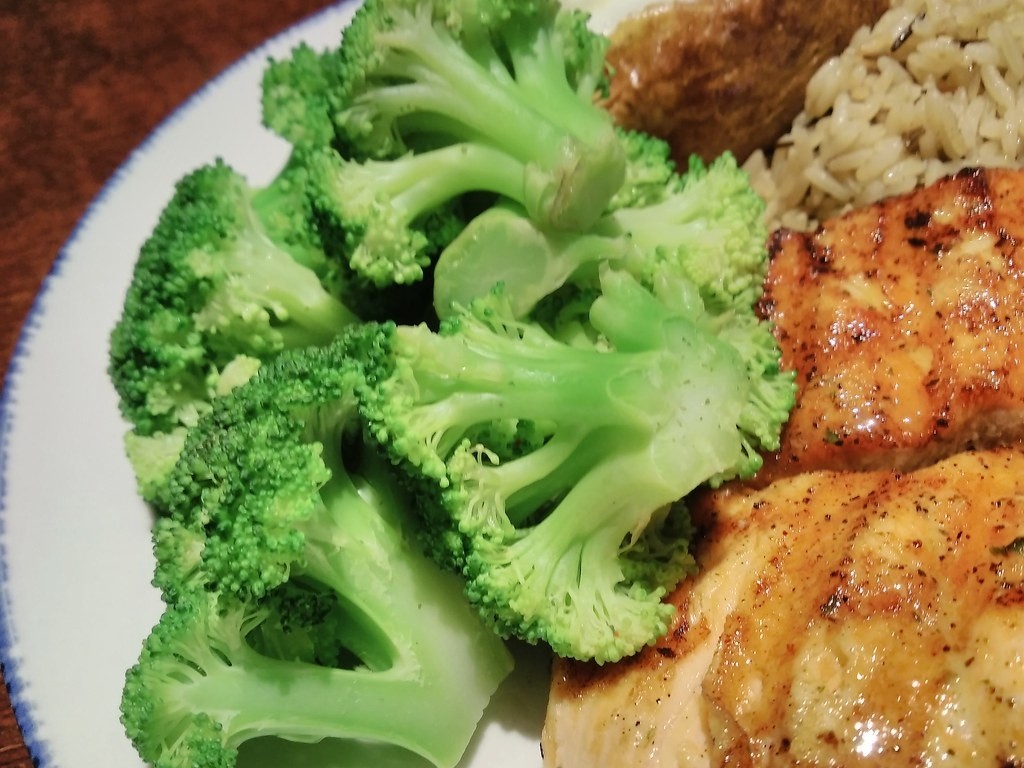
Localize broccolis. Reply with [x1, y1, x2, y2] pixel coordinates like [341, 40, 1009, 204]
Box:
[107, 0, 800, 767]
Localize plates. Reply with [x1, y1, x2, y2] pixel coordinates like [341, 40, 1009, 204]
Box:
[0, 2, 660, 768]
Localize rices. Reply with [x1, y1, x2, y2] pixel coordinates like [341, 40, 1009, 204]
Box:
[742, 0, 1024, 237]
